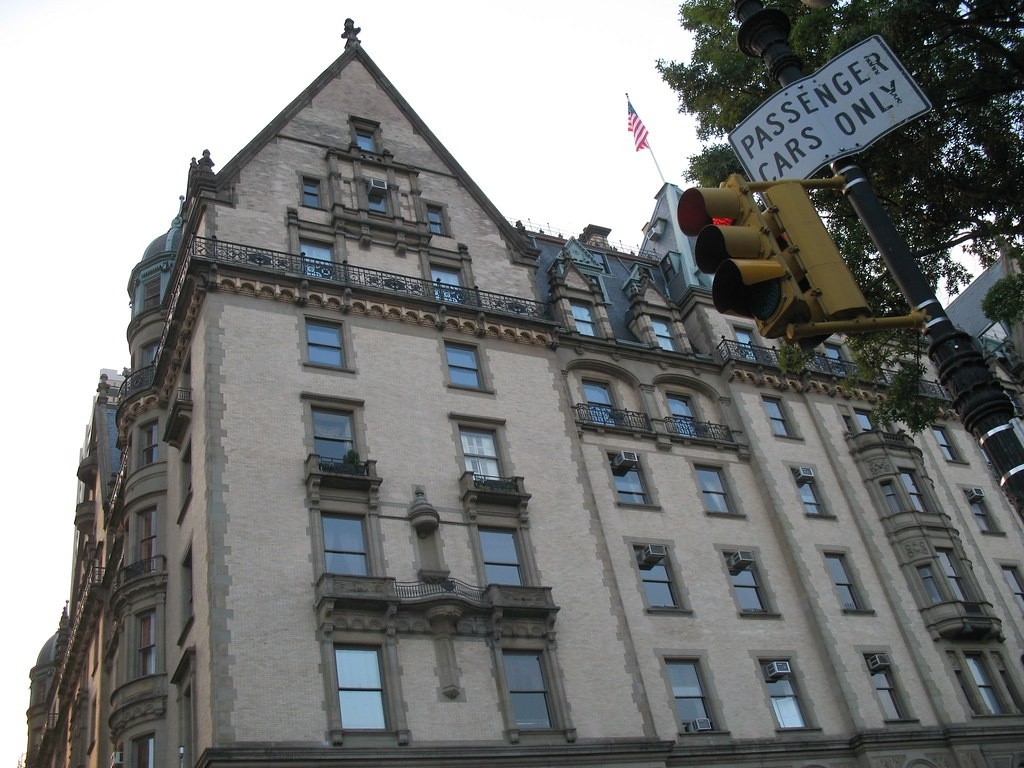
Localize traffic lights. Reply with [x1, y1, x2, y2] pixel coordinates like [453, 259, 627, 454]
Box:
[676, 173, 809, 339]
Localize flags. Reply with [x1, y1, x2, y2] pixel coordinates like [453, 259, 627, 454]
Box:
[627, 101, 649, 152]
